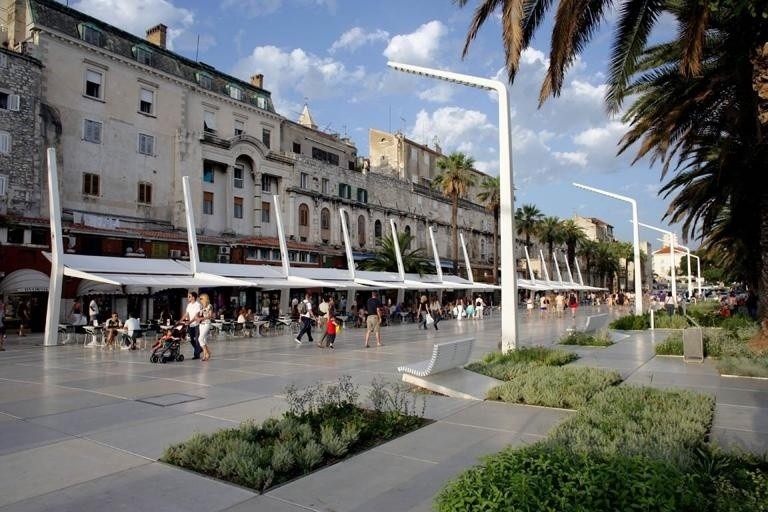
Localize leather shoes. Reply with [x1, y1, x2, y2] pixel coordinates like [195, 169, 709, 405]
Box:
[192, 356, 200, 360]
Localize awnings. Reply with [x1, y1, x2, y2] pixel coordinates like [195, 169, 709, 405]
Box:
[198, 273, 503, 289]
[518, 279, 610, 291]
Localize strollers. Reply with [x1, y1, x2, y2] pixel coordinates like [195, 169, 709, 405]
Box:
[149, 321, 186, 364]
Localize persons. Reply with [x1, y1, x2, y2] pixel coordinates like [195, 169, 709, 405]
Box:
[382, 294, 488, 330]
[519, 288, 675, 320]
[212, 294, 369, 337]
[2, 295, 142, 351]
[365, 290, 386, 347]
[295, 293, 339, 348]
[676, 284, 748, 318]
[147, 292, 214, 362]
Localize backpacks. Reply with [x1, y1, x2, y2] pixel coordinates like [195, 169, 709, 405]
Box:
[477, 302, 481, 306]
[296, 300, 308, 314]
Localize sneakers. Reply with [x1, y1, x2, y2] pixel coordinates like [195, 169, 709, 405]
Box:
[294, 337, 302, 344]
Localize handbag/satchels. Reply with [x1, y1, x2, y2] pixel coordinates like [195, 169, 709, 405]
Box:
[317, 310, 326, 317]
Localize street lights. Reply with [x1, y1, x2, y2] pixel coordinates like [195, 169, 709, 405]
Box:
[385, 60, 520, 356]
[628, 219, 677, 308]
[571, 182, 643, 317]
[673, 248, 702, 301]
[656, 237, 692, 302]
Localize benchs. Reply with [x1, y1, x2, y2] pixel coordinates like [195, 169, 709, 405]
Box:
[567, 311, 611, 339]
[397, 336, 478, 377]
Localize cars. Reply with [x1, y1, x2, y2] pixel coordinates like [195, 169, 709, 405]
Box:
[645, 285, 747, 308]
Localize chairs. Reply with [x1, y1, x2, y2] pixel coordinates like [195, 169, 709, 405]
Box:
[55, 293, 496, 350]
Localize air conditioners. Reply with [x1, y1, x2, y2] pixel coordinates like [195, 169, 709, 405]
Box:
[217, 255, 230, 263]
[170, 249, 181, 258]
[219, 247, 230, 254]
